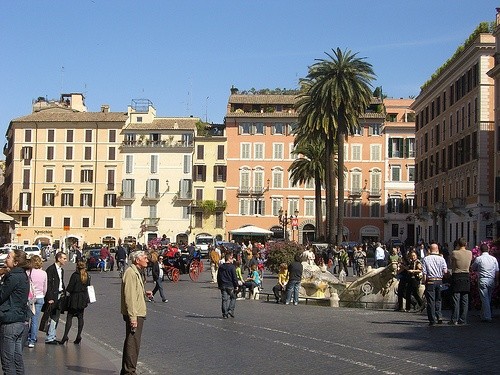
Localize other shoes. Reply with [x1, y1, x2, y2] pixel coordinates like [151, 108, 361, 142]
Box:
[228, 310, 234, 318]
[403, 310, 410, 312]
[420, 305, 425, 312]
[148, 297, 156, 302]
[27, 342, 35, 348]
[210, 280, 217, 283]
[163, 299, 169, 303]
[286, 301, 290, 305]
[437, 320, 443, 324]
[428, 320, 436, 326]
[394, 308, 403, 311]
[294, 302, 298, 305]
[224, 316, 227, 319]
[447, 321, 457, 325]
[413, 303, 417, 310]
[45, 340, 59, 345]
[457, 318, 466, 325]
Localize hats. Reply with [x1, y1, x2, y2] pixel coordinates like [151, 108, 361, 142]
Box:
[159, 256, 163, 259]
[430, 244, 439, 255]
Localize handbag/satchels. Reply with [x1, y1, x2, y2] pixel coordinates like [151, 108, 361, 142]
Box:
[39, 303, 51, 332]
[26, 270, 36, 315]
[415, 262, 423, 281]
[86, 283, 97, 304]
[115, 250, 119, 260]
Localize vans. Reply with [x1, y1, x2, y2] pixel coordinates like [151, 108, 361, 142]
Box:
[194, 236, 216, 257]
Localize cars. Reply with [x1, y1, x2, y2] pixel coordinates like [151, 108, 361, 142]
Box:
[215, 240, 241, 252]
[86, 244, 103, 260]
[0, 243, 43, 268]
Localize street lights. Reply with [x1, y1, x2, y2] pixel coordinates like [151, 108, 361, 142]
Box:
[278, 207, 300, 242]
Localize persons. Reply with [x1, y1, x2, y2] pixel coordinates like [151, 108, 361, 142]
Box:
[45, 252, 67, 344]
[0, 249, 33, 375]
[120, 250, 153, 375]
[471, 244, 499, 323]
[26, 255, 48, 347]
[53, 239, 445, 318]
[447, 237, 473, 326]
[58, 260, 91, 344]
[422, 244, 448, 327]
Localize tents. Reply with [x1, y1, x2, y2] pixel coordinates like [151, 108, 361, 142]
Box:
[228, 225, 272, 241]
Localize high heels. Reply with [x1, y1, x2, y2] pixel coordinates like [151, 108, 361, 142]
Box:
[58, 336, 69, 345]
[73, 335, 82, 345]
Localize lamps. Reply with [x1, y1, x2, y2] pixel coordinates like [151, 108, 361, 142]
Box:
[267, 179, 270, 184]
[166, 180, 169, 186]
[469, 213, 476, 217]
[409, 173, 413, 179]
[54, 184, 57, 190]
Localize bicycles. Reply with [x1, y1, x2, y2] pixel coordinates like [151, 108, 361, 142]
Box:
[118, 261, 125, 279]
